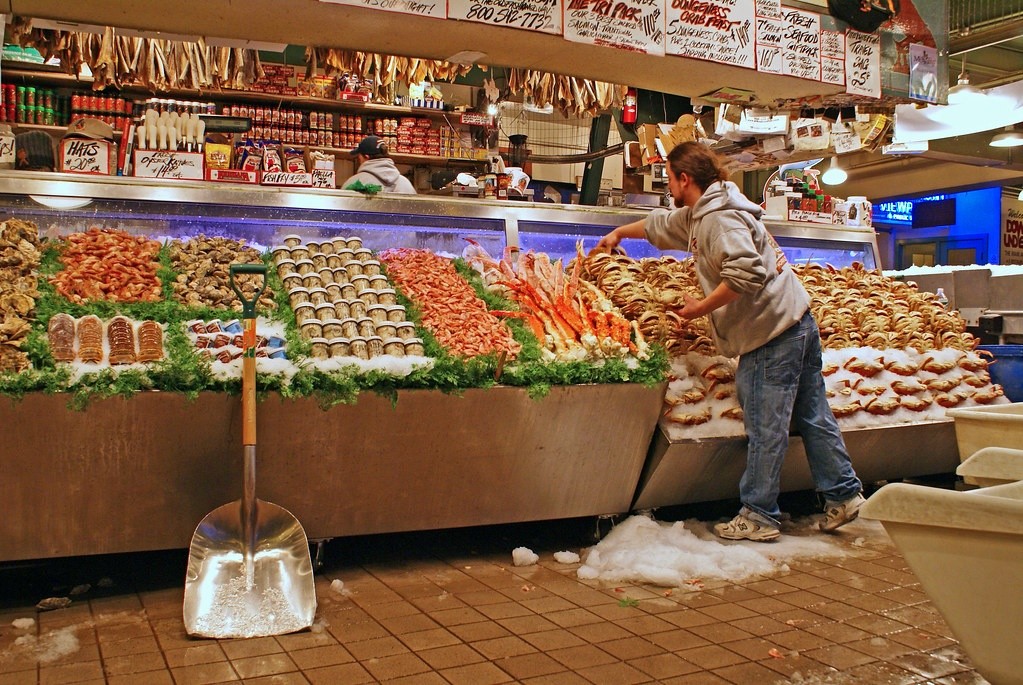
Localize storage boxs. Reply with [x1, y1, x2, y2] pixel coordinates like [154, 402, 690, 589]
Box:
[56, 137, 118, 176]
[310, 169, 337, 189]
[260, 142, 312, 188]
[131, 147, 206, 182]
[204, 167, 261, 184]
[945, 401, 1023, 488]
[832, 210, 847, 226]
[766, 195, 833, 224]
[976, 345, 1023, 403]
[835, 200, 873, 227]
[221, 64, 489, 159]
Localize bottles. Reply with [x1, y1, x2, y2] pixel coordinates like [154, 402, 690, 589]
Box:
[788, 182, 831, 213]
[497, 173, 508, 200]
[478, 172, 485, 198]
[0, 124, 16, 169]
[484, 172, 497, 200]
[936, 288, 948, 307]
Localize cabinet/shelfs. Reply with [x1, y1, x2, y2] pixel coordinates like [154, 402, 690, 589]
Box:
[0, 68, 490, 168]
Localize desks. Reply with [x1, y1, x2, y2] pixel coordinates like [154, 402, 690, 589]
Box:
[427, 186, 535, 202]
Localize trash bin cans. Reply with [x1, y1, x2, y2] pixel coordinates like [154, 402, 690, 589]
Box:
[980, 343, 1023, 400]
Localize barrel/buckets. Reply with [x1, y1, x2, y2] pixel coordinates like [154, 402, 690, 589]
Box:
[976, 345, 1023, 403]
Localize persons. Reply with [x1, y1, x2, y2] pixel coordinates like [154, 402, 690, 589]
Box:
[596, 142, 864, 542]
[341, 137, 416, 194]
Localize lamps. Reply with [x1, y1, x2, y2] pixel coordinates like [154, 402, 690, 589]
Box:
[947, 52, 987, 105]
[822, 156, 847, 186]
[988, 125, 1023, 147]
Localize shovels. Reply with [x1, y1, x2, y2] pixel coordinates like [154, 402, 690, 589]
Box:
[183, 262, 315, 639]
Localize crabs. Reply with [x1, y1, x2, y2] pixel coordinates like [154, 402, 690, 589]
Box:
[852, 379, 887, 396]
[885, 361, 921, 375]
[664, 368, 713, 425]
[956, 353, 1004, 405]
[900, 399, 933, 413]
[821, 364, 865, 417]
[891, 380, 927, 397]
[699, 362, 744, 420]
[920, 355, 977, 408]
[864, 396, 903, 416]
[564, 238, 992, 357]
[843, 357, 885, 377]
[461, 237, 649, 361]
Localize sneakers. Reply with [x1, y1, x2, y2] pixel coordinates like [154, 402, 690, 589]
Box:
[819, 491, 866, 530]
[714, 514, 780, 541]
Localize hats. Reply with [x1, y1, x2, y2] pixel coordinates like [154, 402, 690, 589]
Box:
[349, 136, 388, 157]
[61, 118, 114, 141]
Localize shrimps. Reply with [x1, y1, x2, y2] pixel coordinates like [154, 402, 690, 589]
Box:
[886, 0, 938, 73]
[107, 318, 163, 365]
[47, 313, 103, 364]
[377, 247, 524, 361]
[47, 226, 164, 303]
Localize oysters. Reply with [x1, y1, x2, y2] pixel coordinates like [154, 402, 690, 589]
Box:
[0, 217, 40, 371]
